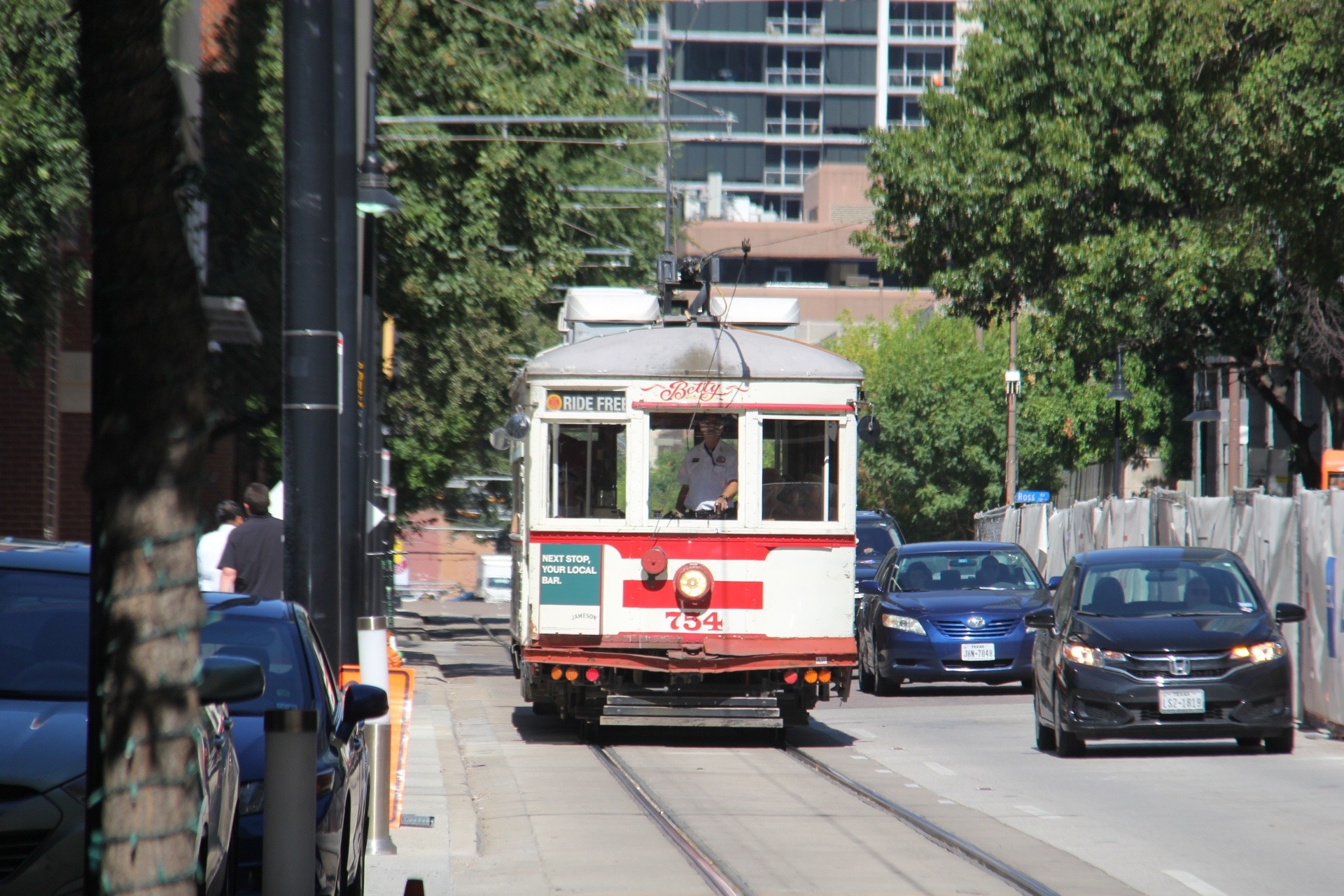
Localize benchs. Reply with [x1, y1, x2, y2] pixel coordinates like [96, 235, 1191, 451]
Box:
[932, 569, 977, 589]
[1126, 600, 1187, 617]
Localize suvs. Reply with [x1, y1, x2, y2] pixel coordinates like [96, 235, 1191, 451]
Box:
[852, 509, 906, 639]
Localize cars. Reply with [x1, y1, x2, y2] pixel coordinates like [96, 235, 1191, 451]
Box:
[858, 541, 1063, 696]
[198, 592, 385, 896]
[1027, 545, 1307, 755]
[0, 538, 266, 896]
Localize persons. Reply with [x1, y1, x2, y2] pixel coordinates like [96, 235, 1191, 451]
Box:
[1180, 576, 1215, 613]
[194, 499, 246, 594]
[672, 413, 739, 518]
[856, 537, 878, 559]
[762, 461, 840, 520]
[973, 554, 1003, 586]
[555, 435, 625, 517]
[905, 560, 933, 592]
[216, 481, 286, 600]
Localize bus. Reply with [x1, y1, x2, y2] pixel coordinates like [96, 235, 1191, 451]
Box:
[513, 240, 867, 737]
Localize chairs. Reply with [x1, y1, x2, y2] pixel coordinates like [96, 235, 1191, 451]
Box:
[904, 568, 932, 590]
[998, 563, 1016, 583]
[1085, 576, 1131, 617]
